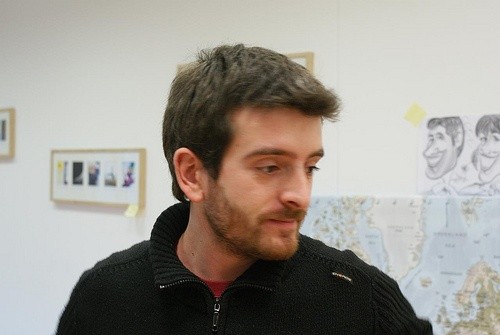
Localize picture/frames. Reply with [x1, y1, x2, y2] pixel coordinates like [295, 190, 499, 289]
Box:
[49, 147, 146, 212]
[0, 106, 16, 162]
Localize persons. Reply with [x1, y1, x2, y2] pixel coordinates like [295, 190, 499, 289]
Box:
[55, 44, 434, 335]
